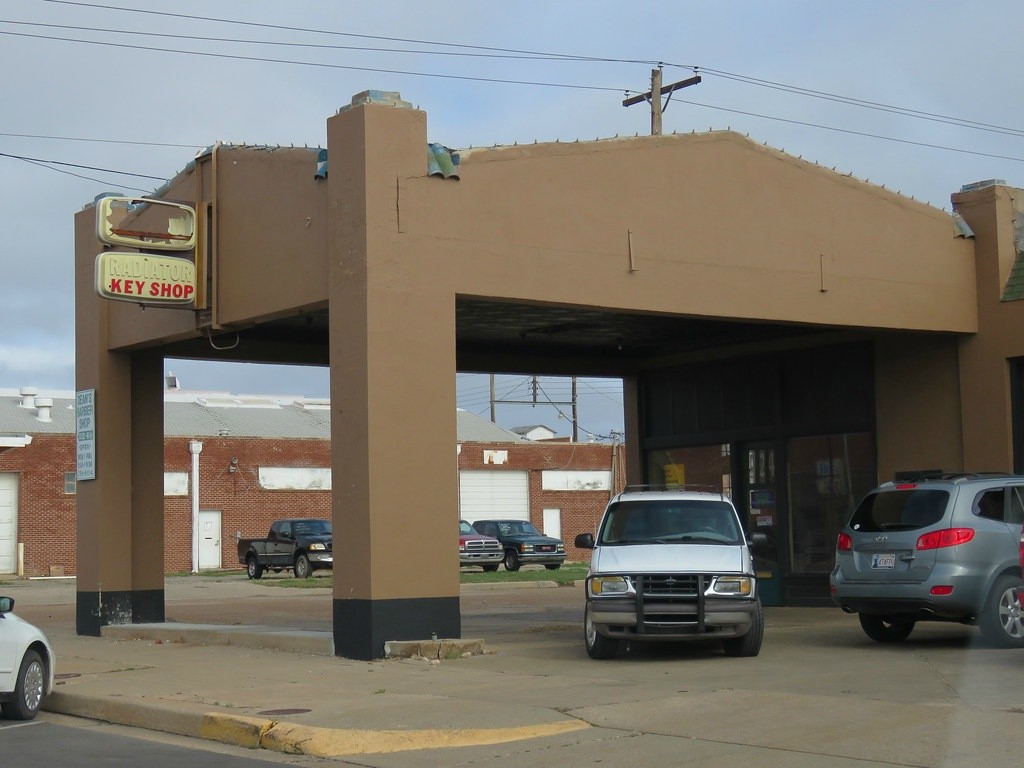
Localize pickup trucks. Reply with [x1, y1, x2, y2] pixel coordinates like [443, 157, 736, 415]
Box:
[237, 518, 334, 580]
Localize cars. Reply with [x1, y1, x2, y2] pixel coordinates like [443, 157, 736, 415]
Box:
[0, 593, 55, 720]
[458, 519, 506, 573]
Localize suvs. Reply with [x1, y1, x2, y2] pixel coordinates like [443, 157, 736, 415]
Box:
[471, 519, 567, 571]
[575, 484, 772, 661]
[828, 469, 1024, 642]
